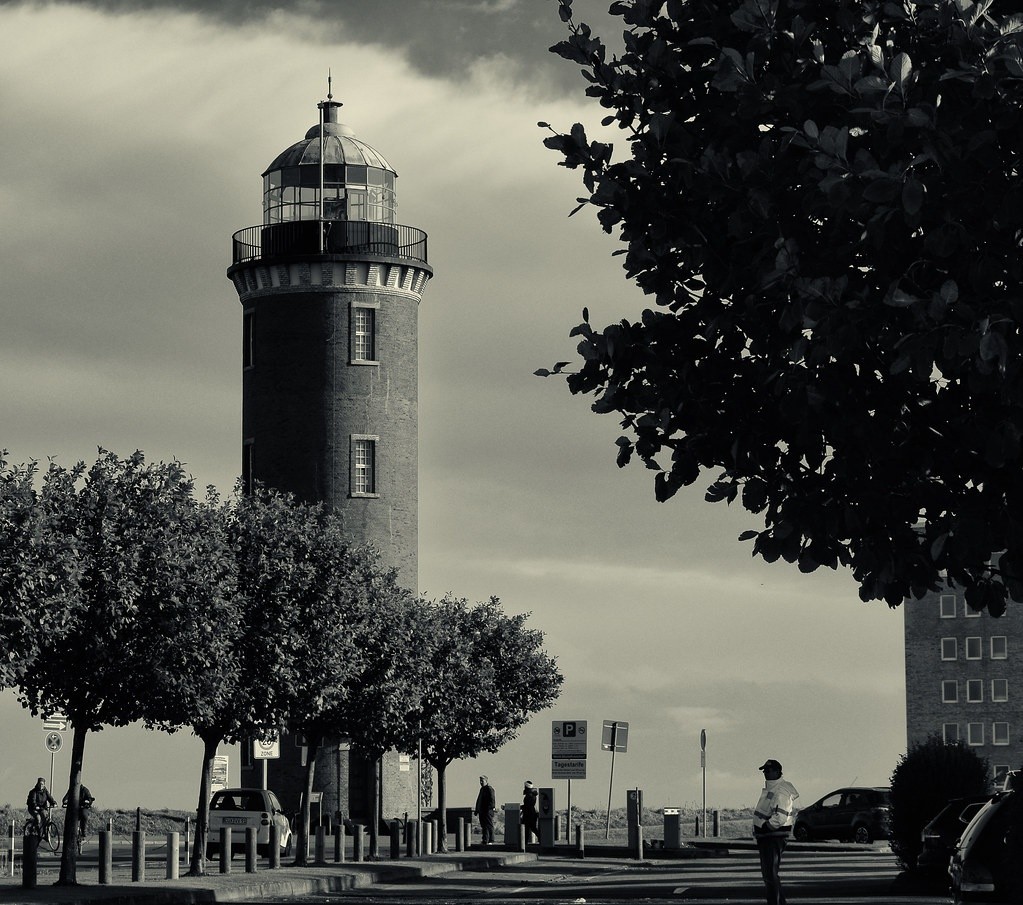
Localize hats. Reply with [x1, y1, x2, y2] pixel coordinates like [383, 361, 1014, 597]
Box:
[478, 774, 489, 781]
[759, 758, 783, 770]
[37, 776, 45, 782]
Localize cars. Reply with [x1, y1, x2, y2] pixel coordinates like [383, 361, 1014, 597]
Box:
[914, 769, 1023, 905]
[203, 787, 293, 860]
[793, 786, 894, 844]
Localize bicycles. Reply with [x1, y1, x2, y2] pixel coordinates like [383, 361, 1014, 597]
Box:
[62, 801, 91, 855]
[24, 804, 60, 852]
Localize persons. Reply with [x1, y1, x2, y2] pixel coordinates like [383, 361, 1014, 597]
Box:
[62, 784, 94, 843]
[474, 775, 496, 844]
[521, 780, 540, 844]
[27, 777, 57, 839]
[752, 759, 800, 904]
